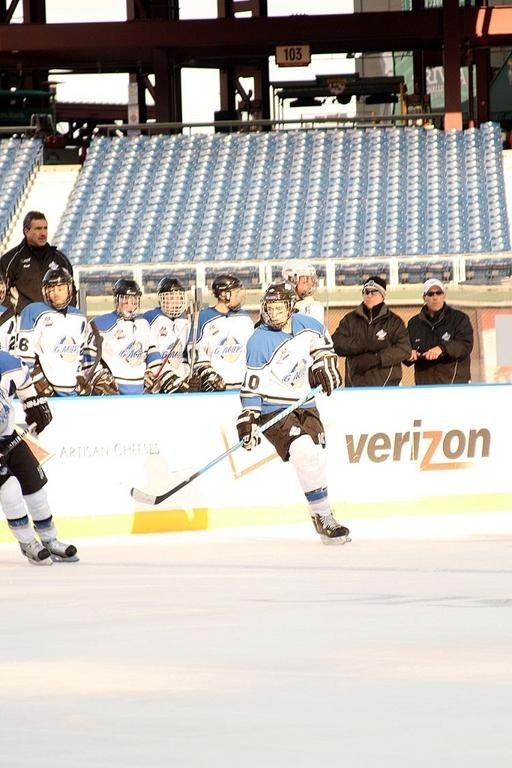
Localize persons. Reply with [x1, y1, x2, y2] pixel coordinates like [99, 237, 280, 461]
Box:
[331, 275, 411, 386]
[404, 279, 473, 384]
[86, 278, 179, 395]
[234, 279, 351, 539]
[1, 389, 56, 565]
[0, 274, 20, 357]
[19, 266, 96, 397]
[282, 258, 325, 326]
[0, 348, 80, 565]
[143, 277, 192, 393]
[189, 275, 256, 390]
[0, 211, 77, 314]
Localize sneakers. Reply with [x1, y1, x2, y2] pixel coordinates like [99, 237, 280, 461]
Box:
[42, 538, 77, 558]
[19, 539, 51, 562]
[311, 514, 349, 536]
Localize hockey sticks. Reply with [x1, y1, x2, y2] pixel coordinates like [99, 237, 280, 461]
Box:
[77, 321, 103, 396]
[131, 385, 327, 506]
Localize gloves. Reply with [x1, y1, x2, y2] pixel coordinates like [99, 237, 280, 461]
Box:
[309, 340, 387, 396]
[24, 363, 120, 434]
[237, 407, 262, 450]
[149, 361, 225, 393]
[353, 354, 381, 372]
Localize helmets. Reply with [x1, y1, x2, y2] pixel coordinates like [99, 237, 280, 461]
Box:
[41, 268, 73, 311]
[113, 259, 318, 330]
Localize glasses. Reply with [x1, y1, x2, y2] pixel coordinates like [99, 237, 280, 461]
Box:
[363, 288, 378, 296]
[426, 291, 442, 296]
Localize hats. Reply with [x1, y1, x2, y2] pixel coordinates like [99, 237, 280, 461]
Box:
[363, 277, 386, 298]
[423, 279, 444, 295]
